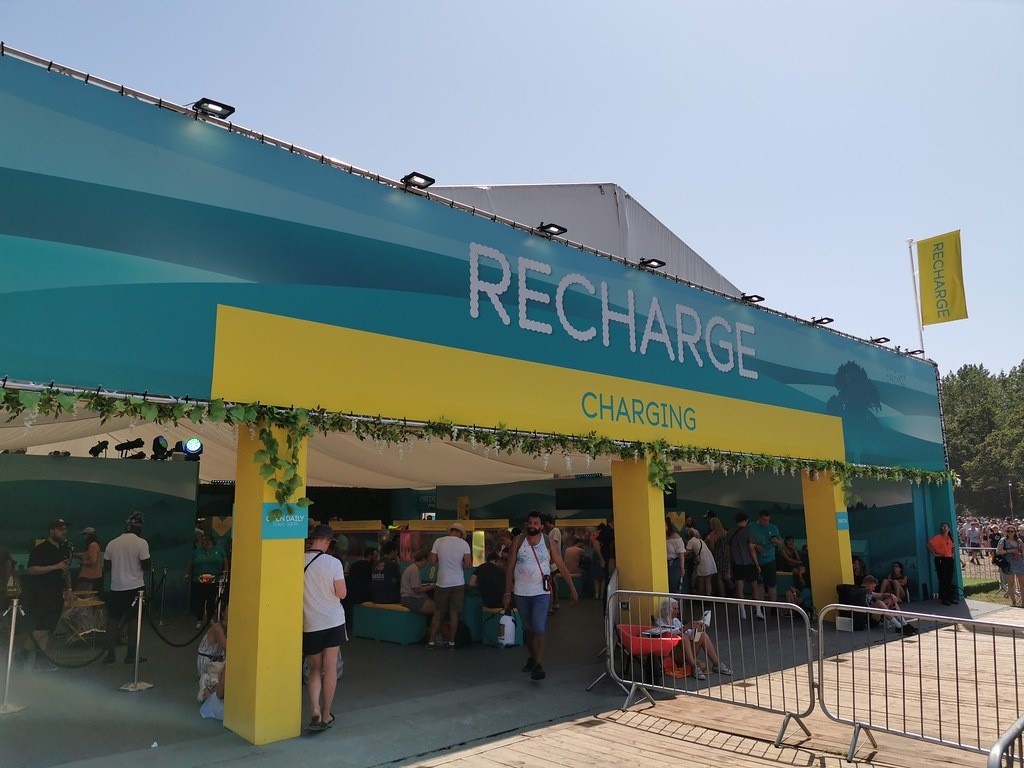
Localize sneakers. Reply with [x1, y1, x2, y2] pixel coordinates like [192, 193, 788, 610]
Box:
[425, 642, 435, 651]
[713, 662, 733, 675]
[446, 643, 455, 651]
[691, 667, 706, 680]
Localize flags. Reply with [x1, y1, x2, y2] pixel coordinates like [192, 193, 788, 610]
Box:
[916, 231, 971, 327]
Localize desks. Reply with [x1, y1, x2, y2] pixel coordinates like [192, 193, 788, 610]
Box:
[54, 589, 106, 649]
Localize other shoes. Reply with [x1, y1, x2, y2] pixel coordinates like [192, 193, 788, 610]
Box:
[970, 560, 980, 565]
[198, 665, 218, 704]
[740, 612, 748, 619]
[14, 652, 58, 672]
[125, 656, 147, 664]
[943, 599, 958, 606]
[522, 658, 545, 680]
[196, 622, 202, 629]
[756, 612, 764, 620]
[895, 623, 918, 635]
[103, 655, 116, 664]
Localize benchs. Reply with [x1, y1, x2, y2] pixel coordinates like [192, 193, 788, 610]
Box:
[353, 602, 426, 644]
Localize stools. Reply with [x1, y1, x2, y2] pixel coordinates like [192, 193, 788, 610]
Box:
[775, 571, 794, 593]
[556, 573, 584, 599]
[482, 605, 524, 645]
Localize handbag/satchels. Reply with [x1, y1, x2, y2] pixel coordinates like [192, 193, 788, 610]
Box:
[303, 647, 344, 684]
[950, 584, 965, 600]
[992, 555, 1010, 574]
[454, 620, 472, 647]
[690, 554, 700, 565]
[197, 574, 217, 584]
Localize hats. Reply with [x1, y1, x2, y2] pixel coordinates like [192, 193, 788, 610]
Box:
[448, 523, 466, 538]
[310, 525, 337, 543]
[48, 517, 71, 528]
[80, 527, 96, 535]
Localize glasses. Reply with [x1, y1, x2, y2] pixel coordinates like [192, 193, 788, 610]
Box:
[1007, 530, 1014, 533]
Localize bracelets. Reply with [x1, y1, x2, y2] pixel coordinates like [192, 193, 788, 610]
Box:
[65, 588, 73, 591]
[1006, 550, 1009, 554]
[504, 592, 512, 597]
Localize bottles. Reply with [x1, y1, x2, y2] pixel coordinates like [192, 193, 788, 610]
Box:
[932, 593, 935, 599]
[936, 592, 939, 599]
[1014, 548, 1018, 560]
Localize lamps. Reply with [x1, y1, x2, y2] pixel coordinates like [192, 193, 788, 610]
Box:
[194, 98, 235, 119]
[872, 337, 890, 343]
[910, 350, 924, 355]
[539, 223, 568, 236]
[641, 259, 666, 268]
[815, 318, 834, 325]
[741, 294, 765, 302]
[401, 171, 435, 190]
[90, 436, 204, 462]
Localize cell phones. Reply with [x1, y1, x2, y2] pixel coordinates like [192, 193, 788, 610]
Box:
[769, 536, 776, 540]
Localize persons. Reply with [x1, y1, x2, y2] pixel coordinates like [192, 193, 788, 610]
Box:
[996, 525, 1024, 608]
[927, 523, 960, 606]
[665, 509, 812, 621]
[853, 559, 868, 585]
[502, 510, 578, 680]
[195, 517, 206, 541]
[14, 518, 74, 673]
[371, 541, 401, 604]
[562, 523, 615, 600]
[73, 527, 103, 599]
[400, 550, 452, 646]
[303, 525, 347, 731]
[861, 575, 919, 635]
[347, 547, 380, 602]
[0, 547, 12, 633]
[544, 514, 562, 609]
[497, 527, 523, 566]
[197, 596, 227, 677]
[198, 663, 225, 720]
[655, 597, 734, 680]
[957, 515, 1024, 568]
[880, 562, 908, 603]
[425, 523, 472, 651]
[184, 534, 229, 629]
[102, 510, 151, 666]
[469, 553, 505, 609]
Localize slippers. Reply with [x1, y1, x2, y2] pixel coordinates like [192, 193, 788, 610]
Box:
[304, 712, 335, 730]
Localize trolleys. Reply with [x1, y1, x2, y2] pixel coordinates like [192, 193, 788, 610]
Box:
[614, 614, 682, 687]
